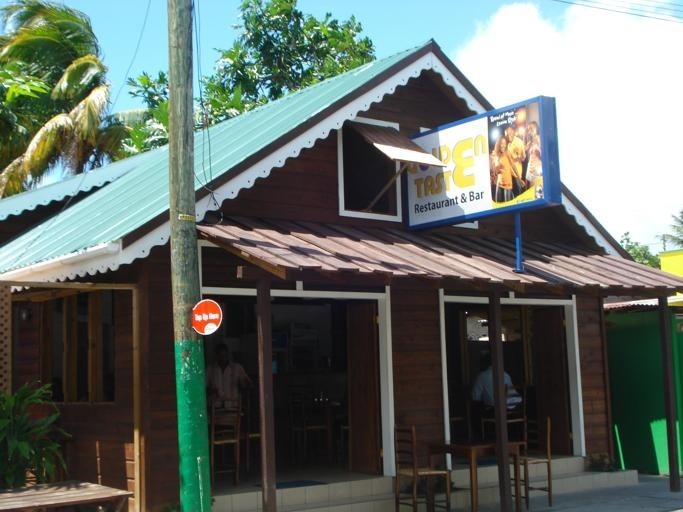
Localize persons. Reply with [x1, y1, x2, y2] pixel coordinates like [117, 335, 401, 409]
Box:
[471, 357, 523, 418]
[489, 121, 542, 203]
[206, 345, 253, 408]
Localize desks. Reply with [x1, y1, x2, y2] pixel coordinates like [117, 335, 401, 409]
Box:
[428, 440, 523, 509]
[0, 480, 134, 512]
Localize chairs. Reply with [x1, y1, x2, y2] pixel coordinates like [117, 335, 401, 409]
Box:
[394, 424, 451, 511]
[482, 384, 529, 461]
[209, 396, 353, 485]
[500, 418, 553, 508]
